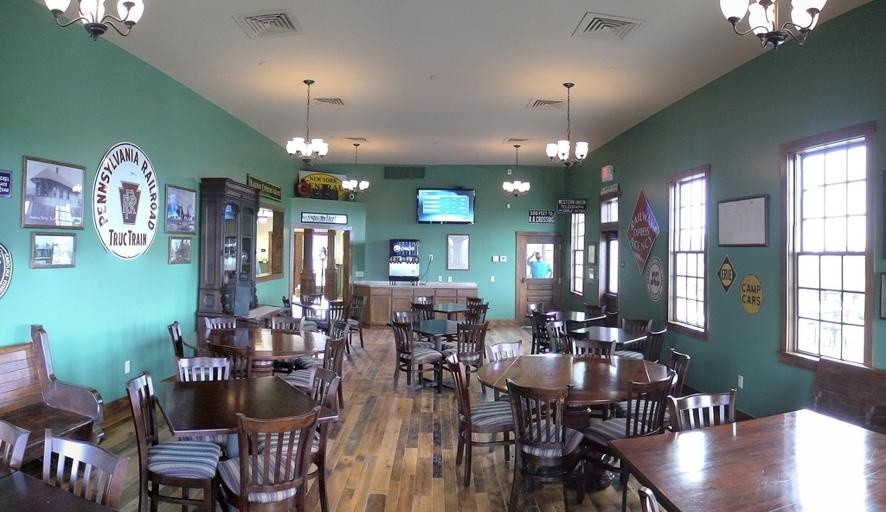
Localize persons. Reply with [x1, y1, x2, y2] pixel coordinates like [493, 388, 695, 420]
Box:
[527, 251, 553, 278]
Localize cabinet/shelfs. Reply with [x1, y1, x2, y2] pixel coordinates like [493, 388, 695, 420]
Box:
[195, 175, 261, 320]
[352, 278, 476, 326]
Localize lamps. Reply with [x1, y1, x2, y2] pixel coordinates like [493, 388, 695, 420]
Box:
[501, 141, 531, 197]
[284, 78, 332, 159]
[543, 79, 595, 167]
[341, 139, 375, 198]
[37, 0, 145, 42]
[717, 0, 828, 50]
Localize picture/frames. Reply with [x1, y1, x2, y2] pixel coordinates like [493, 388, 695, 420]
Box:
[28, 231, 75, 270]
[715, 191, 771, 251]
[161, 182, 196, 236]
[246, 170, 281, 202]
[165, 241, 191, 265]
[16, 152, 86, 230]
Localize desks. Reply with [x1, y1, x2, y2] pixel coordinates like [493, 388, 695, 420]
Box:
[607, 407, 885, 512]
[0, 464, 118, 512]
[148, 377, 341, 511]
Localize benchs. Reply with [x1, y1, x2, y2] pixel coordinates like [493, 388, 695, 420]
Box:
[0, 326, 107, 483]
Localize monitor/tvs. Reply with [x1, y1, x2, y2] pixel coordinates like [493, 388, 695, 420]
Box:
[416, 188, 476, 225]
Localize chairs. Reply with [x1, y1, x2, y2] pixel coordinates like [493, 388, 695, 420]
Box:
[177, 354, 227, 458]
[445, 350, 526, 484]
[525, 302, 665, 364]
[167, 293, 366, 410]
[126, 376, 223, 512]
[250, 366, 341, 512]
[392, 294, 491, 394]
[610, 349, 691, 462]
[506, 379, 585, 511]
[571, 337, 615, 421]
[219, 406, 321, 511]
[485, 340, 534, 460]
[44, 426, 123, 503]
[0, 417, 36, 470]
[667, 387, 739, 432]
[143, 371, 225, 456]
[580, 370, 675, 512]
